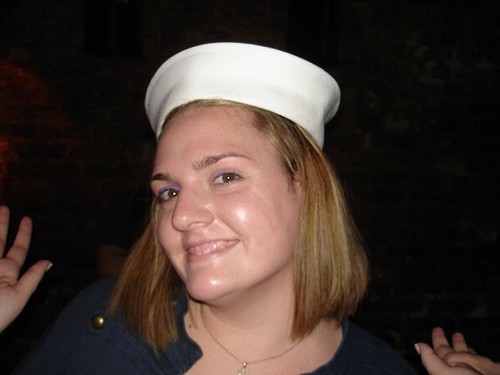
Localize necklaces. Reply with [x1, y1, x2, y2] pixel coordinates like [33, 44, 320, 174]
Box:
[199, 302, 307, 375]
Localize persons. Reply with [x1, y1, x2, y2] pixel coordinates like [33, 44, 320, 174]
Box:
[95, 186, 153, 277]
[1, 42, 500, 375]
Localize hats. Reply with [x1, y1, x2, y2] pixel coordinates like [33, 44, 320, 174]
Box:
[143, 41, 342, 152]
